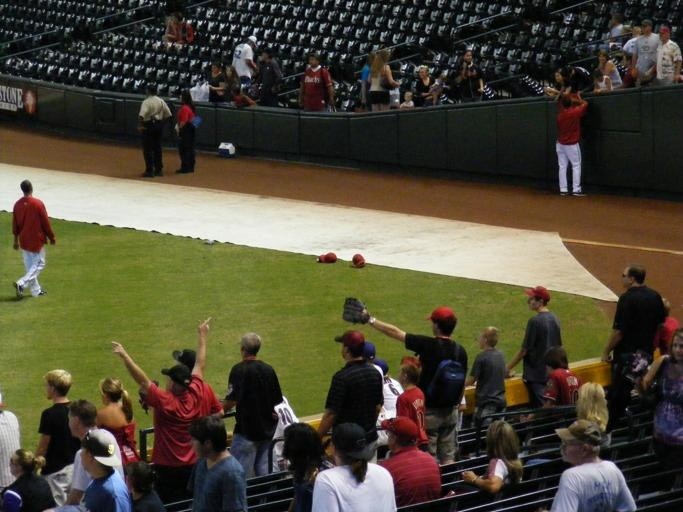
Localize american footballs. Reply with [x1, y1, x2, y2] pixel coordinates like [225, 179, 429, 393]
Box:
[326, 253, 337, 262]
[353, 254, 365, 267]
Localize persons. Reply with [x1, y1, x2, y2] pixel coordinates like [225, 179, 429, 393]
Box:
[0, 317, 397, 512]
[138, 85, 171, 178]
[342, 264, 683, 511]
[456, 50, 486, 105]
[556, 96, 588, 197]
[299, 53, 335, 113]
[543, 48, 622, 98]
[178, 21, 194, 44]
[361, 49, 444, 112]
[176, 90, 197, 173]
[161, 16, 179, 43]
[209, 35, 283, 108]
[606, 14, 682, 89]
[13, 180, 56, 299]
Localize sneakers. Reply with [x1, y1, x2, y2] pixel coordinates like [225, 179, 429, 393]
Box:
[12, 281, 24, 299]
[560, 192, 589, 197]
[37, 291, 46, 295]
[142, 168, 192, 177]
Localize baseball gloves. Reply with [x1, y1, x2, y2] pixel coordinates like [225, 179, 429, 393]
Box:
[343, 297, 370, 324]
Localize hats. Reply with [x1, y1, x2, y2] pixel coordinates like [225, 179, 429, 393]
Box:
[161, 365, 192, 386]
[554, 419, 603, 446]
[173, 349, 196, 368]
[640, 18, 652, 26]
[427, 307, 457, 324]
[87, 429, 122, 467]
[525, 285, 551, 302]
[659, 27, 671, 35]
[249, 35, 257, 47]
[318, 252, 337, 263]
[381, 417, 418, 444]
[335, 330, 388, 376]
[333, 423, 374, 461]
[351, 253, 364, 267]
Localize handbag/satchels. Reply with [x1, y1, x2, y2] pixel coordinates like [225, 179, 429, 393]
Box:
[639, 381, 659, 409]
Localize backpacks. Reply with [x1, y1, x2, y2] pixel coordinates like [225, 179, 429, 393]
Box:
[425, 337, 466, 407]
